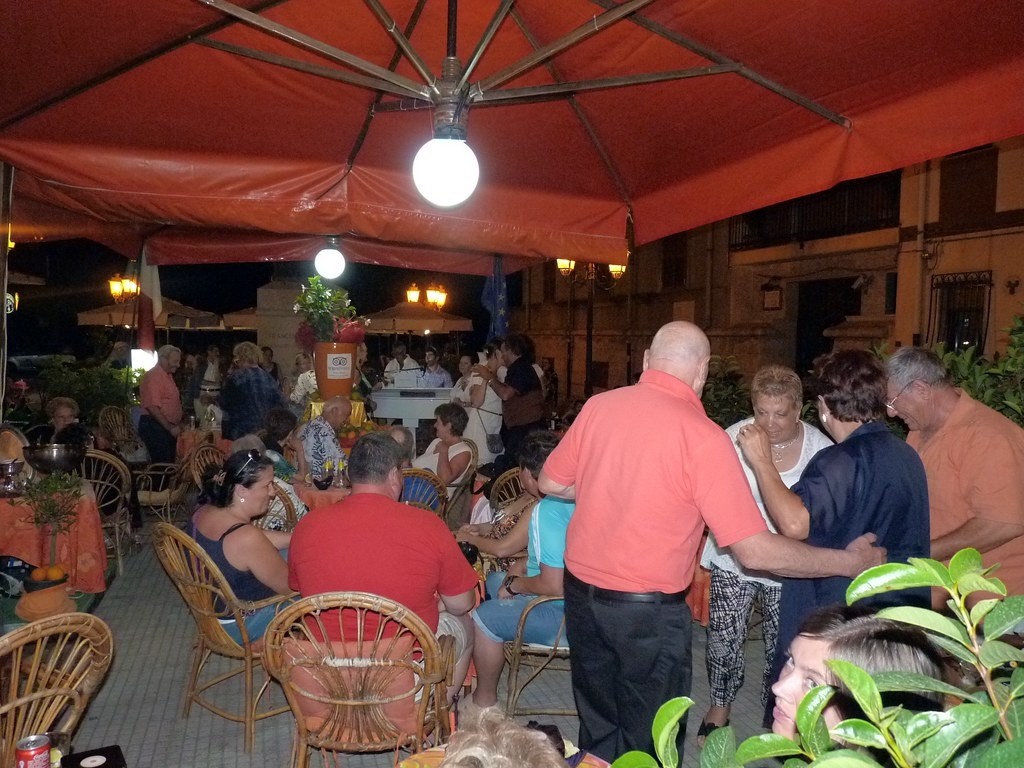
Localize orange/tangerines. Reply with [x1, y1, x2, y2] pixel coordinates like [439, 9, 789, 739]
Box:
[32, 563, 68, 580]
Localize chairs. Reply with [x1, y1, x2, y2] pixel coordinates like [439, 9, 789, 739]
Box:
[402, 437, 579, 723]
[150, 520, 301, 755]
[0, 611, 113, 768]
[0, 405, 296, 575]
[262, 591, 456, 768]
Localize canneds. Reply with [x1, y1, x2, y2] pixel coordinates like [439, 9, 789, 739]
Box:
[14, 735, 51, 768]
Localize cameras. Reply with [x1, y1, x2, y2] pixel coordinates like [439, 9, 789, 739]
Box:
[456, 541, 478, 565]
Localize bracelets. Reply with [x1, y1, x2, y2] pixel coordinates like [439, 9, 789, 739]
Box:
[463, 401, 465, 406]
[488, 378, 501, 391]
[506, 575, 519, 595]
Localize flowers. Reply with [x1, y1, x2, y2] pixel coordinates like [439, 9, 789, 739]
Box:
[292, 273, 372, 342]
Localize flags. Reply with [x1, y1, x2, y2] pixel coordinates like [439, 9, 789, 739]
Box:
[484, 257, 507, 344]
[140, 250, 162, 320]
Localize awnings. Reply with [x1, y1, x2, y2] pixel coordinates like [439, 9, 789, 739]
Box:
[223, 308, 256, 329]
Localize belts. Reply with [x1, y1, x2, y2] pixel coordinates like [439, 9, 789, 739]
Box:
[564, 566, 686, 605]
[200, 387, 222, 392]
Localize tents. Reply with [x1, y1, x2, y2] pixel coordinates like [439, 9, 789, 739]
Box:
[0, 0, 1024, 398]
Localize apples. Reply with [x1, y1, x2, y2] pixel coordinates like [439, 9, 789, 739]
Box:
[338, 420, 374, 438]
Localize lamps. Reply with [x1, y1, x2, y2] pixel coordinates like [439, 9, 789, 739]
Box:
[413, 57, 479, 207]
[314, 234, 346, 279]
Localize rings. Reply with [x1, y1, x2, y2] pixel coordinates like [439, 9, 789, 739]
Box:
[742, 429, 748, 434]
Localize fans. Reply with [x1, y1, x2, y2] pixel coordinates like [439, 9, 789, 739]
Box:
[1004, 274, 1022, 297]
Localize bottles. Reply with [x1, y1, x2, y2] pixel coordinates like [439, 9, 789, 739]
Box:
[324, 457, 350, 487]
[191, 416, 195, 428]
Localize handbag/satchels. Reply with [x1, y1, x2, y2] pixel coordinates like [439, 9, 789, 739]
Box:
[485, 432, 504, 452]
[470, 454, 522, 502]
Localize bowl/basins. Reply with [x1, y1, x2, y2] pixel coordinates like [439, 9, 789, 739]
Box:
[22, 444, 86, 474]
[313, 476, 332, 490]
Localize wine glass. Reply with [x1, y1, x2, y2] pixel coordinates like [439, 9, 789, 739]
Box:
[0, 459, 24, 491]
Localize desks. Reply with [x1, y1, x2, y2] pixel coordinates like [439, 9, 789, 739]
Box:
[175, 427, 234, 456]
[0, 477, 109, 592]
[301, 399, 367, 427]
[292, 478, 354, 511]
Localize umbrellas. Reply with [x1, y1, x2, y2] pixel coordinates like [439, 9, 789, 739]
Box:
[77, 295, 224, 346]
[364, 302, 472, 356]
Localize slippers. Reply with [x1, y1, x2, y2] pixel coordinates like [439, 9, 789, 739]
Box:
[696, 718, 732, 751]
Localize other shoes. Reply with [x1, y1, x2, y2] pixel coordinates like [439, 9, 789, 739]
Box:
[457, 689, 502, 721]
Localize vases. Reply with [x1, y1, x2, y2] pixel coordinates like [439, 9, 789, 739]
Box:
[312, 342, 357, 399]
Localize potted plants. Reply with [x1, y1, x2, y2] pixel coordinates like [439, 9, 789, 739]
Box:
[31, 354, 145, 451]
[6, 468, 86, 593]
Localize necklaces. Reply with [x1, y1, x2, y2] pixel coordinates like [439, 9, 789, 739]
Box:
[772, 423, 800, 461]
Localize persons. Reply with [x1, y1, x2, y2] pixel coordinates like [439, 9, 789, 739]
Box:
[356, 336, 546, 468]
[438, 706, 569, 768]
[537, 321, 887, 767]
[1, 342, 318, 557]
[737, 348, 931, 729]
[771, 606, 944, 768]
[183, 395, 576, 750]
[886, 346, 1023, 636]
[698, 361, 834, 750]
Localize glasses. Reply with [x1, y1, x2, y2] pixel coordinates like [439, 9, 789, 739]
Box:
[883, 377, 931, 410]
[235, 447, 262, 477]
[55, 414, 74, 422]
[234, 359, 241, 365]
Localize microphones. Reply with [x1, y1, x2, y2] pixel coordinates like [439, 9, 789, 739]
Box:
[423, 363, 427, 375]
